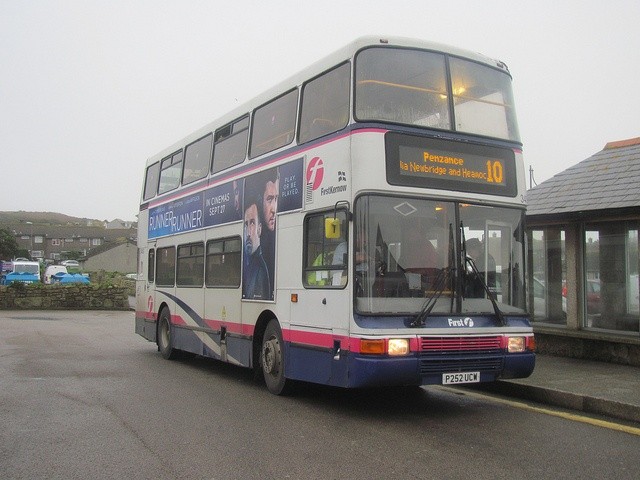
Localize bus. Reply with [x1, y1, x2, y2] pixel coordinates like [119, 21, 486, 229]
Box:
[136, 33, 536, 396]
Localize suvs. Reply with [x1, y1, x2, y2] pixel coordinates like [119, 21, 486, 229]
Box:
[466, 266, 567, 321]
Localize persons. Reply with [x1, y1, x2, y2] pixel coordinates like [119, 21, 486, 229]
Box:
[463, 237, 497, 299]
[259, 177, 278, 300]
[244, 198, 271, 300]
[397, 226, 436, 284]
[331, 228, 369, 287]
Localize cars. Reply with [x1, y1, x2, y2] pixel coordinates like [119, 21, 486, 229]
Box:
[44, 265, 67, 285]
[108, 273, 138, 310]
[561, 279, 600, 314]
[61, 260, 79, 266]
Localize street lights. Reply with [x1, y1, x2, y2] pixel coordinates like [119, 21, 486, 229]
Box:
[27, 220, 33, 255]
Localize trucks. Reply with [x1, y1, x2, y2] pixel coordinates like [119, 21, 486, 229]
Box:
[13, 261, 40, 283]
